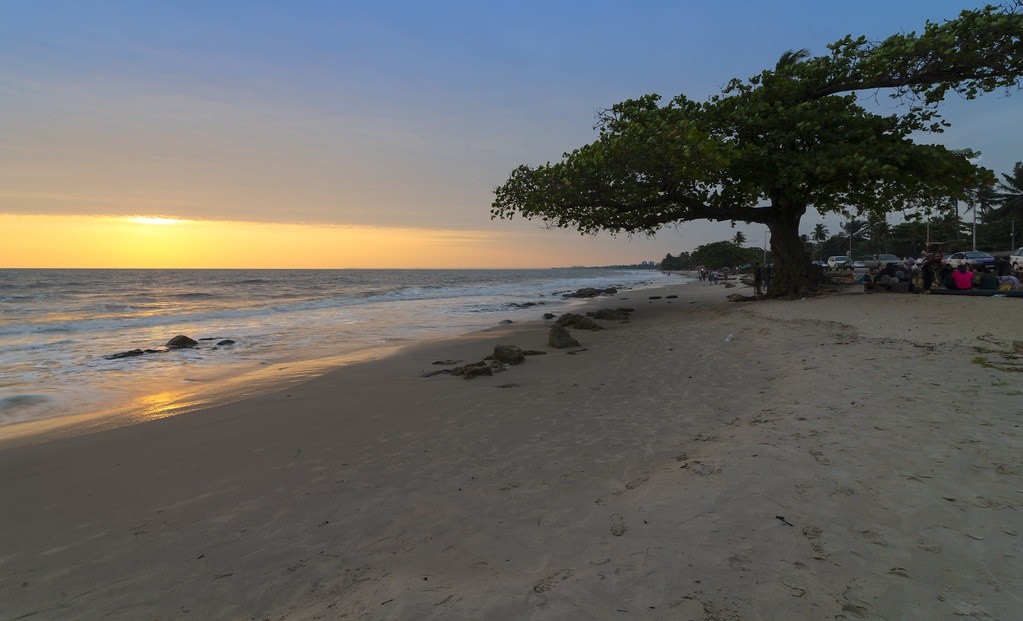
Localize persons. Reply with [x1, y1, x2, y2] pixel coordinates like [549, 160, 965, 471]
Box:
[753, 261, 763, 297]
[764, 264, 773, 295]
[698, 267, 731, 286]
[874, 246, 1023, 294]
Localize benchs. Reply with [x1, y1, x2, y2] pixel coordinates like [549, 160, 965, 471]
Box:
[930, 288, 1023, 296]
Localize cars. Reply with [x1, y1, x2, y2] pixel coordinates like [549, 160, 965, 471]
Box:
[1009, 247, 1023, 273]
[944, 250, 996, 272]
[811, 253, 901, 272]
